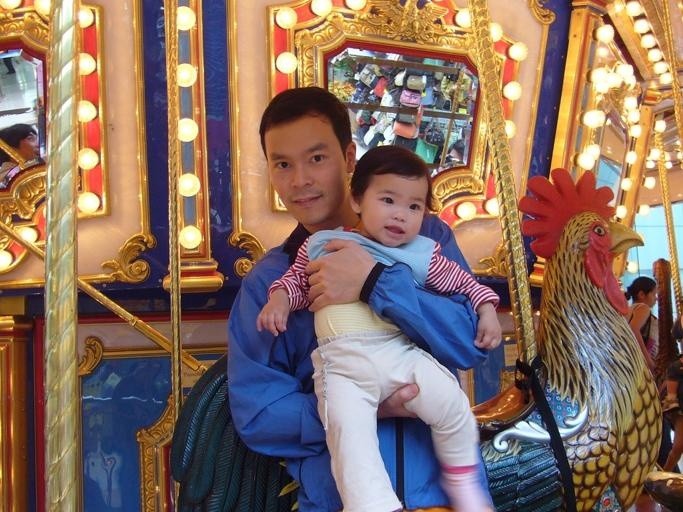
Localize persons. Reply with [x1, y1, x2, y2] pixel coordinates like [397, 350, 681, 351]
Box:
[255, 145, 505, 511]
[625, 276, 680, 473]
[226, 87, 493, 511]
[0, 124, 41, 183]
[662, 315, 683, 413]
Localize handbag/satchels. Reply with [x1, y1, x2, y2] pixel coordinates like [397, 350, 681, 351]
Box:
[349, 51, 479, 164]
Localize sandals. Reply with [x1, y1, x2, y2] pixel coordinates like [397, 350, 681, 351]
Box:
[661, 395, 680, 415]
